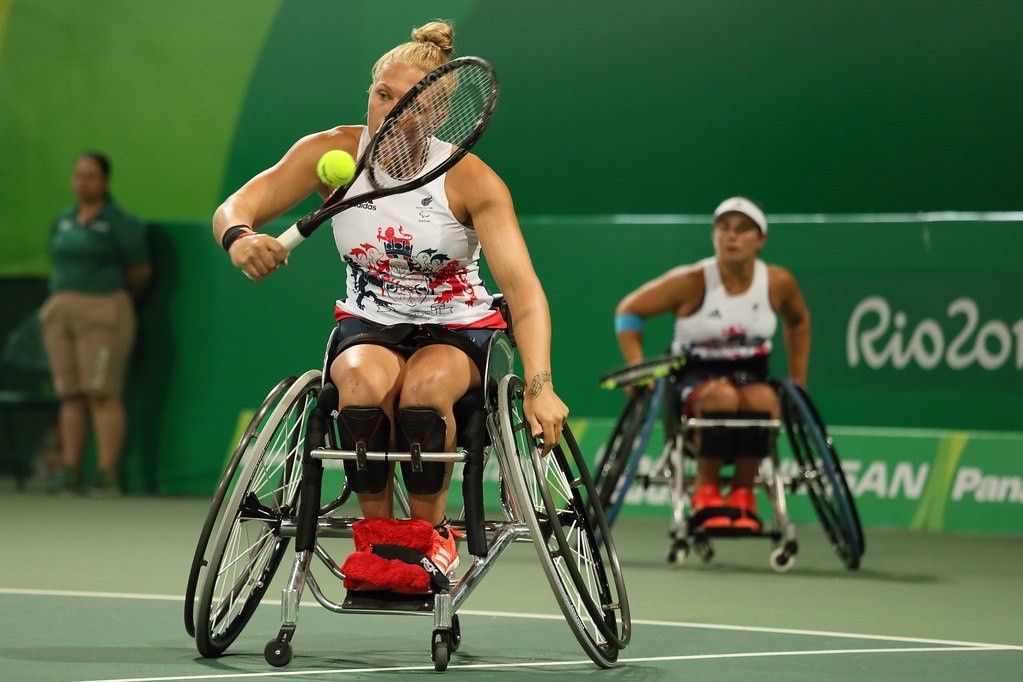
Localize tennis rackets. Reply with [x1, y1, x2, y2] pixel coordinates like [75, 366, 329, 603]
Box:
[238, 56, 500, 282]
[598, 355, 689, 395]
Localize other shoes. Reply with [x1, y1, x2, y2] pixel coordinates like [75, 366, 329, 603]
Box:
[88, 472, 122, 500]
[48, 468, 84, 494]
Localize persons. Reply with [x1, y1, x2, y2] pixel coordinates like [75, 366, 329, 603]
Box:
[616, 196, 810, 531]
[38, 153, 151, 496]
[214, 21, 568, 592]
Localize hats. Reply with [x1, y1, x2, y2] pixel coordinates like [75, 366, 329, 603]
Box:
[712, 197, 768, 236]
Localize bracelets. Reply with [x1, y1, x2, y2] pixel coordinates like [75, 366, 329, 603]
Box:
[617, 313, 643, 335]
[222, 224, 256, 251]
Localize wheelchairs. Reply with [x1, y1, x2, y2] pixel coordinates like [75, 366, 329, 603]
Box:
[576, 374, 867, 574]
[183, 294, 631, 670]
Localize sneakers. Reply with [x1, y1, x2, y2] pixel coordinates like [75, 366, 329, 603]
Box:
[726, 486, 761, 534]
[692, 483, 731, 534]
[428, 526, 466, 594]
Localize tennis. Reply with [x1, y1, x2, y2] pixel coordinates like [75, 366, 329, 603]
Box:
[316, 150, 357, 186]
